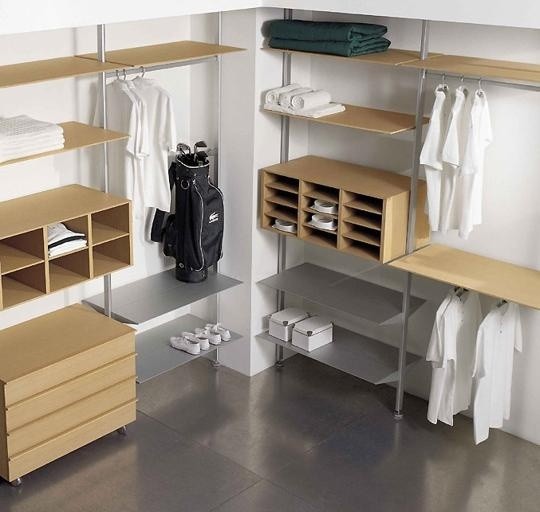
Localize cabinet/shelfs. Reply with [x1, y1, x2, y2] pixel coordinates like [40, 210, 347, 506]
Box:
[261, 9, 539, 420]
[0, 24, 248, 485]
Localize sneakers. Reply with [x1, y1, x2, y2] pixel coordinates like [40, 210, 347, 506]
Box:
[170, 323, 232, 355]
